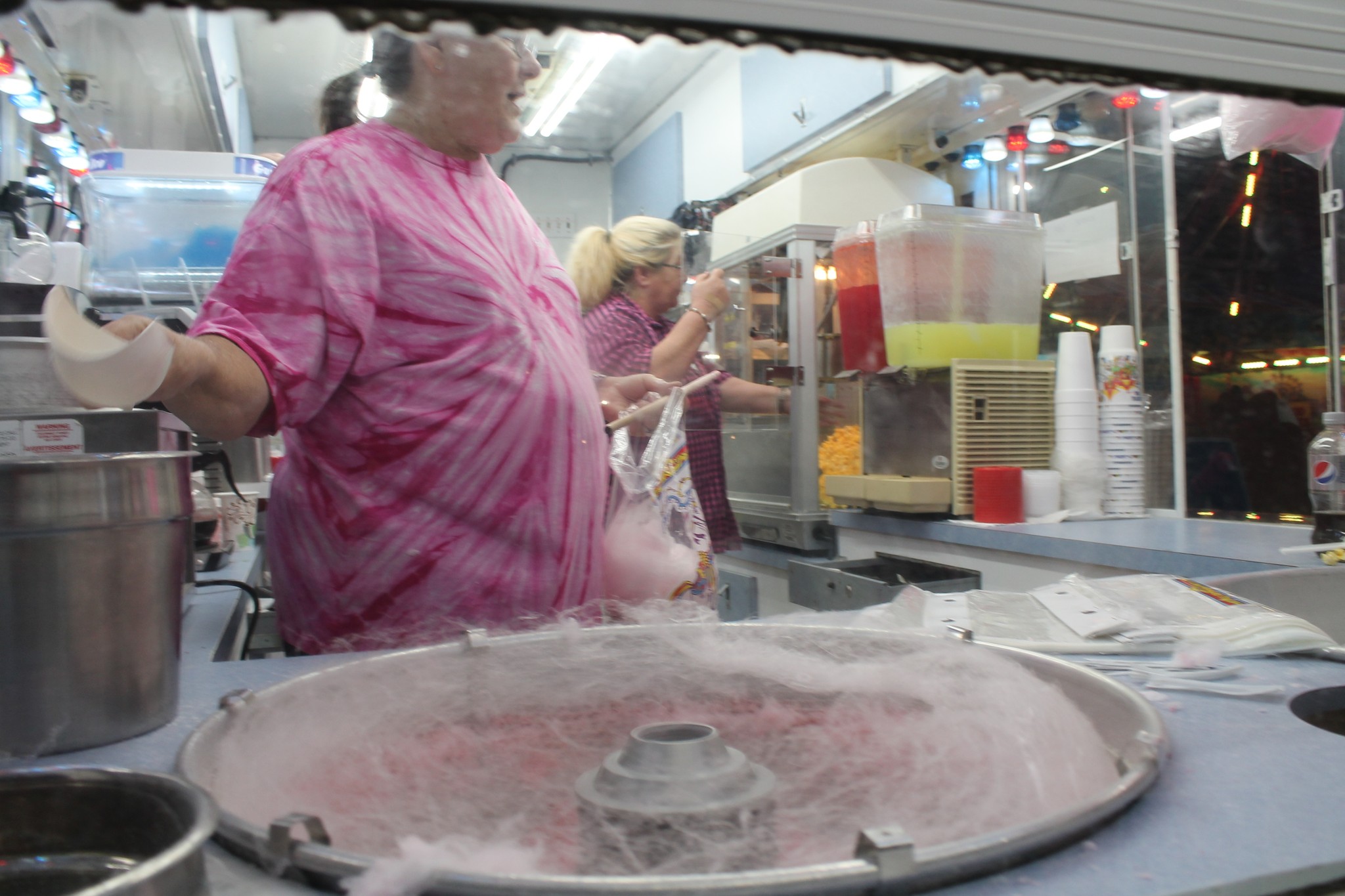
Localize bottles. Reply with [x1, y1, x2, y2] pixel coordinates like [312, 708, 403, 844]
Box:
[1306, 410, 1345, 546]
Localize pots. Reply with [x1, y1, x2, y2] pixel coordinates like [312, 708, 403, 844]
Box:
[1, 396, 201, 764]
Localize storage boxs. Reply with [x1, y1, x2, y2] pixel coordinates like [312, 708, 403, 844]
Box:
[873, 204, 1044, 369]
[832, 219, 885, 373]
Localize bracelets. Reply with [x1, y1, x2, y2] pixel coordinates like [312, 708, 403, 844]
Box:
[684, 307, 713, 332]
[776, 388, 789, 414]
[590, 369, 609, 390]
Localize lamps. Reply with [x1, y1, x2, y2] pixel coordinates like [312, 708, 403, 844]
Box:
[959, 80, 1169, 171]
[0, 37, 91, 177]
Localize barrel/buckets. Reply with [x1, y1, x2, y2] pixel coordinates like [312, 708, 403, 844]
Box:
[1, 761, 212, 896]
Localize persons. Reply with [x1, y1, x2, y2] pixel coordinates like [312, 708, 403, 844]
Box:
[561, 215, 850, 558]
[101, 22, 681, 665]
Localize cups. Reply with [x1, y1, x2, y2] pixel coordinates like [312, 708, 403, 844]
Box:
[1053, 323, 1145, 524]
